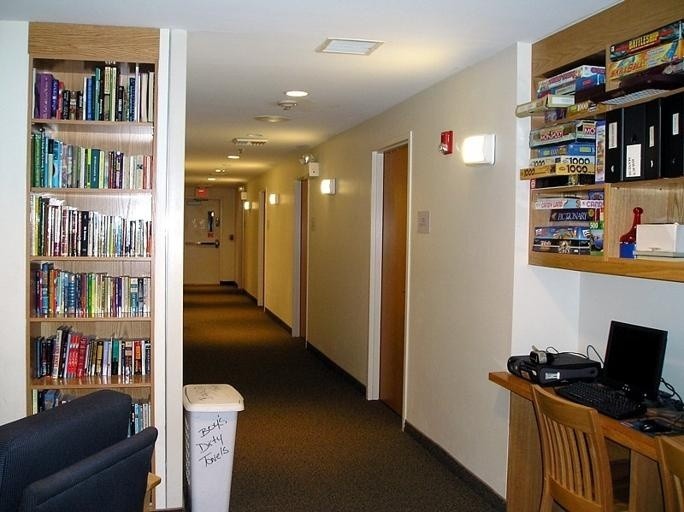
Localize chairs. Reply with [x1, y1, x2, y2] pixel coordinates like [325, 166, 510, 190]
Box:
[526, 383, 684, 512]
[2, 388, 159, 510]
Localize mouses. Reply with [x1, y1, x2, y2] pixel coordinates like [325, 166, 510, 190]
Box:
[641, 418, 658, 433]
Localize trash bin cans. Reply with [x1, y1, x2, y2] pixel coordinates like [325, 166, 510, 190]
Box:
[183, 384, 246, 512]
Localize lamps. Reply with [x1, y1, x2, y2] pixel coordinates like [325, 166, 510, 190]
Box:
[319, 178, 337, 195]
[268, 192, 280, 209]
[456, 133, 497, 167]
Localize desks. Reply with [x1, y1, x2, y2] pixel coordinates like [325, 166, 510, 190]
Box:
[486, 368, 684, 512]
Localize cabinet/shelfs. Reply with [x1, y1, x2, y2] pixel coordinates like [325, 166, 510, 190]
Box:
[3, 21, 170, 512]
[516, 0, 684, 284]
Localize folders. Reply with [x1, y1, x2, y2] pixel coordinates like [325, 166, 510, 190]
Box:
[605, 89, 684, 183]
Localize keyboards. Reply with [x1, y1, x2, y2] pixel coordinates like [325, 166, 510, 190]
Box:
[554, 380, 648, 422]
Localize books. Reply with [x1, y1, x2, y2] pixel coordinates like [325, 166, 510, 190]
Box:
[127, 400, 151, 438]
[30, 132, 153, 190]
[32, 63, 154, 122]
[34, 325, 152, 377]
[30, 262, 150, 318]
[33, 388, 75, 415]
[28, 194, 152, 258]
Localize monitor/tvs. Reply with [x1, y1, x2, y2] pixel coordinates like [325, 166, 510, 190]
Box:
[600, 319, 668, 401]
